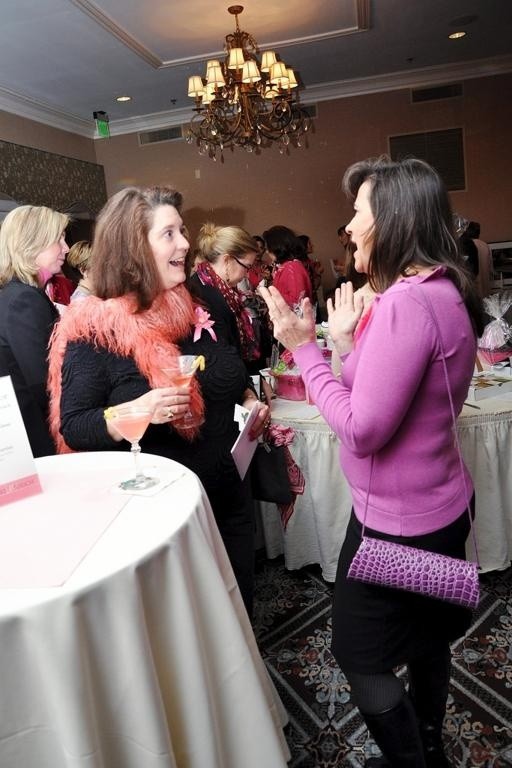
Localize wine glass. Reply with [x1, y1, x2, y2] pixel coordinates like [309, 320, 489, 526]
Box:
[161, 355, 207, 430]
[105, 406, 157, 490]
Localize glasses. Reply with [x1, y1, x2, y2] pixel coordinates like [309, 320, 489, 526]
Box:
[233, 255, 251, 273]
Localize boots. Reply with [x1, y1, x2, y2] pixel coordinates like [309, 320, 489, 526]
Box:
[408, 687, 447, 768]
[364, 696, 426, 768]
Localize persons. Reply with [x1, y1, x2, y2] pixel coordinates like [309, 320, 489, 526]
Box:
[256, 158, 476, 768]
[2, 206, 70, 456]
[189, 225, 368, 406]
[45, 241, 94, 314]
[47, 185, 271, 552]
[452, 221, 495, 336]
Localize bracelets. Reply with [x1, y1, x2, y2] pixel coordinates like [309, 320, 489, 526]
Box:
[244, 393, 256, 397]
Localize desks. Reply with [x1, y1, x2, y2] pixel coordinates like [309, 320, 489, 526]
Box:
[247, 363, 512, 584]
[0, 449, 293, 768]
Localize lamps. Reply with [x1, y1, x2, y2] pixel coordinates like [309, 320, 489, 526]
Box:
[180, 6, 313, 164]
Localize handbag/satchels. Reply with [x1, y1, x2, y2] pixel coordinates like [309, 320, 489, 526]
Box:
[347, 536, 479, 609]
[251, 442, 291, 505]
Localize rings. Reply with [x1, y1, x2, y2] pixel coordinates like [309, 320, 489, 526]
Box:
[262, 421, 268, 430]
[167, 409, 174, 419]
